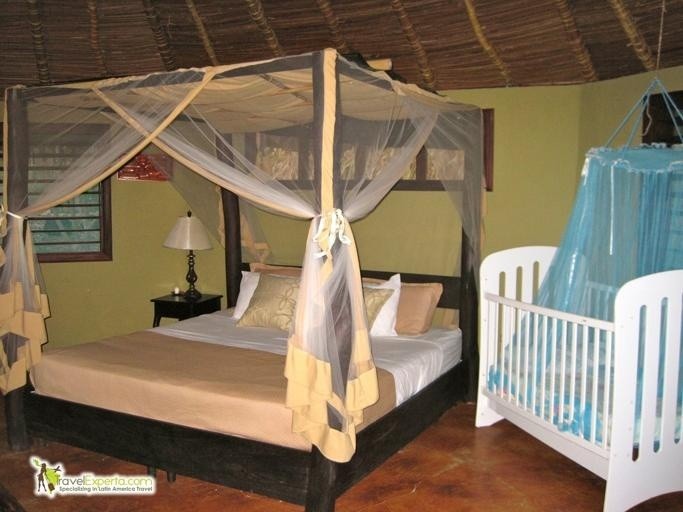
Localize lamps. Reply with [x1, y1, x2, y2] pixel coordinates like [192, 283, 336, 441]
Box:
[163, 210, 212, 298]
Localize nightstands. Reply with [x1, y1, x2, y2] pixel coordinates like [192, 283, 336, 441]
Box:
[149, 292, 223, 327]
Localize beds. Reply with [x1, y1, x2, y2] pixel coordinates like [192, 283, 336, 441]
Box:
[475, 245, 683, 512]
[27, 261, 474, 512]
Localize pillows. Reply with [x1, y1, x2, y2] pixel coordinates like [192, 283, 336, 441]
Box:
[231, 263, 443, 337]
